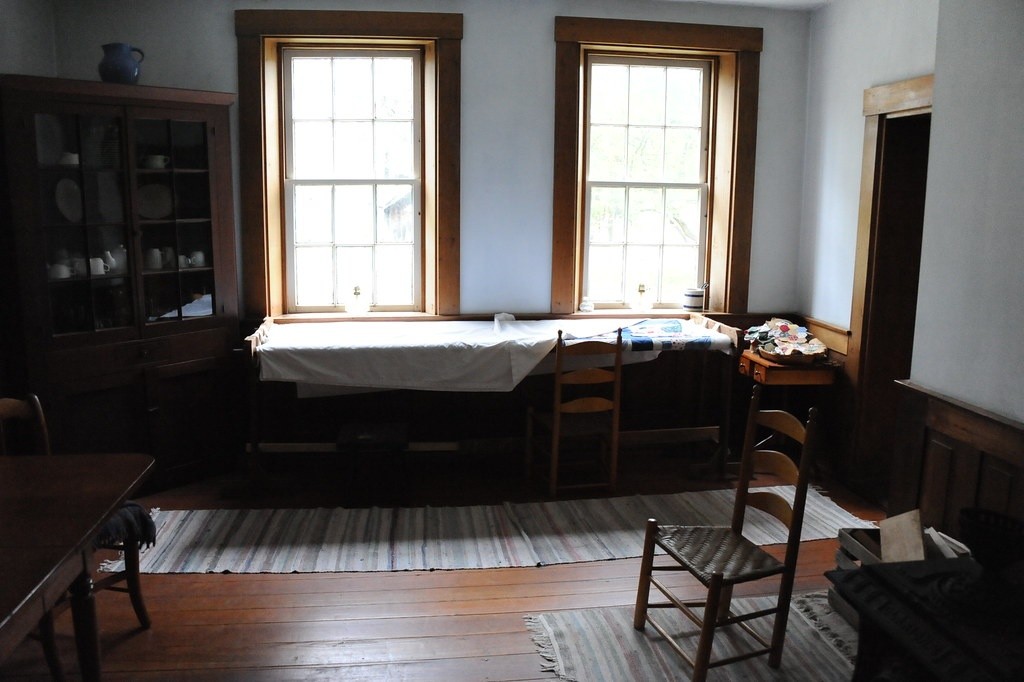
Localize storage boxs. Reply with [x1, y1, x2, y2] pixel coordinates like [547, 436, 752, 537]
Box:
[829, 519, 968, 630]
[879, 509, 942, 561]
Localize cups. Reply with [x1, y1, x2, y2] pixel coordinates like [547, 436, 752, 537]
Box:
[51, 264, 76, 278]
[74, 258, 87, 276]
[190, 251, 205, 267]
[179, 255, 191, 268]
[90, 258, 110, 275]
[683, 288, 704, 311]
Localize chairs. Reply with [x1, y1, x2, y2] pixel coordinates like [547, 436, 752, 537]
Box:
[0, 388, 51, 458]
[631, 379, 824, 679]
[550, 327, 626, 487]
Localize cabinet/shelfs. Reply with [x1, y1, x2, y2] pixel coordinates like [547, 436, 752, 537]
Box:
[0, 74, 240, 496]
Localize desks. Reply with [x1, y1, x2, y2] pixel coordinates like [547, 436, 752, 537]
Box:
[253, 317, 742, 460]
[0, 452, 156, 682]
[723, 350, 841, 445]
[827, 559, 1024, 682]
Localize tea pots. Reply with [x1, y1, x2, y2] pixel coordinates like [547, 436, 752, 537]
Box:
[106, 244, 126, 274]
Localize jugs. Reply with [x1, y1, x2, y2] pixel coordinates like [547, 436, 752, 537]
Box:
[98, 42, 144, 85]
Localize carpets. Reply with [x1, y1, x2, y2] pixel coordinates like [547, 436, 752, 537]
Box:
[526, 591, 859, 682]
[100, 484, 875, 574]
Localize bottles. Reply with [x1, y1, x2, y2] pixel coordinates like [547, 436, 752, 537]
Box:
[152, 249, 162, 269]
